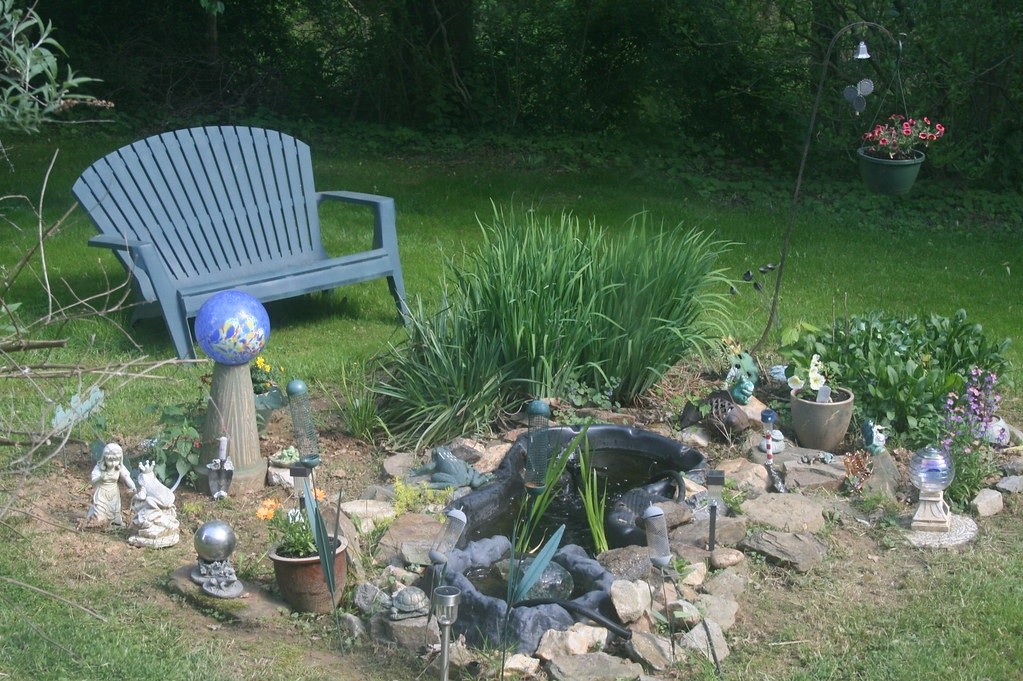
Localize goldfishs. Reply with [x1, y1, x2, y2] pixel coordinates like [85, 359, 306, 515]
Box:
[678, 390, 752, 447]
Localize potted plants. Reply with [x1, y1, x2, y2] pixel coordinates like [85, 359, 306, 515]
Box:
[268, 446, 316, 490]
[266, 509, 348, 616]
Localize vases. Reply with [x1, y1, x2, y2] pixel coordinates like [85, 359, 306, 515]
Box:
[254, 389, 283, 431]
[857, 146, 925, 196]
[790, 387, 854, 451]
[980, 416, 1010, 447]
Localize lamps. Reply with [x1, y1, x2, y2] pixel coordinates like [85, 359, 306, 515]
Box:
[909, 443, 955, 532]
[852, 25, 871, 59]
[433, 585, 462, 681]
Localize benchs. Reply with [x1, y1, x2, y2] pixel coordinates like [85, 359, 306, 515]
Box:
[71, 126, 410, 365]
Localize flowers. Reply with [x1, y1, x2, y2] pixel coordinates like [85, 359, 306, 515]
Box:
[250, 355, 287, 394]
[862, 114, 944, 160]
[788, 353, 843, 402]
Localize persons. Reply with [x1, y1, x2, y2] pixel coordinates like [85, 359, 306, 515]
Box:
[86, 443, 137, 528]
